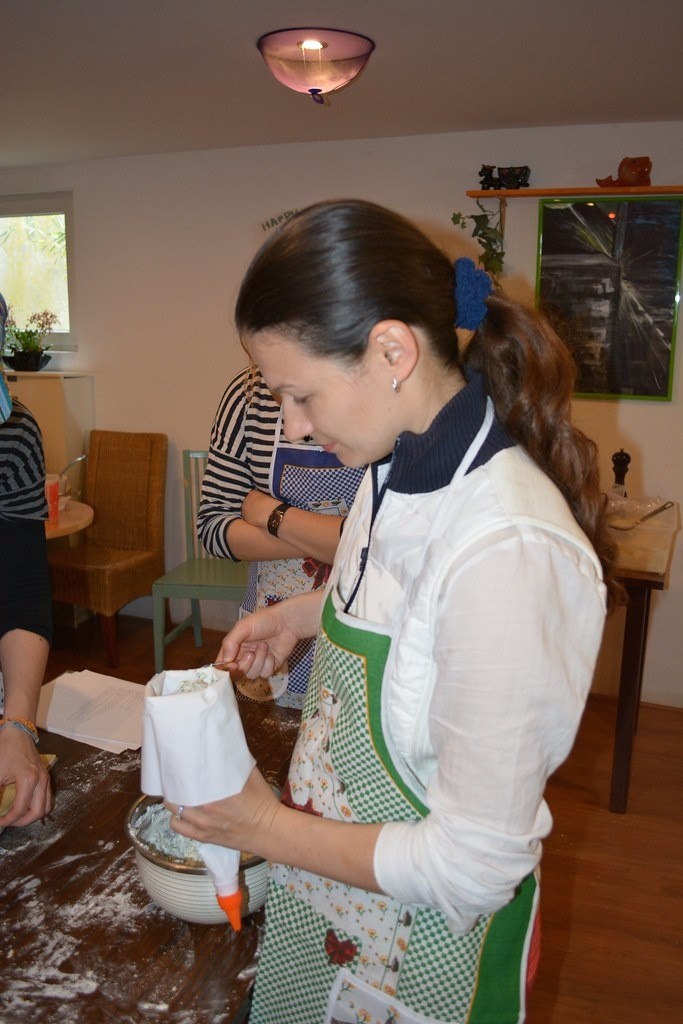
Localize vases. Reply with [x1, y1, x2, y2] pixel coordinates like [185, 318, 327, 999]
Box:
[2, 354, 52, 372]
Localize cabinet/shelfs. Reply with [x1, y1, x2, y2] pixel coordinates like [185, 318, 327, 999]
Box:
[3, 369, 94, 502]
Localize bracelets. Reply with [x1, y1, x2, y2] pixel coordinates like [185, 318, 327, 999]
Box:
[0, 717, 40, 745]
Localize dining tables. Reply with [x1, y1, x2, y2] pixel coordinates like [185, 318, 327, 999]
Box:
[0, 702, 303, 1024]
[45, 499, 94, 542]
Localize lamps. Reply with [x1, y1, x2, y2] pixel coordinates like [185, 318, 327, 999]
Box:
[255, 27, 375, 105]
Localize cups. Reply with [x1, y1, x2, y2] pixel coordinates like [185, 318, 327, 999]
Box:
[43, 474, 59, 525]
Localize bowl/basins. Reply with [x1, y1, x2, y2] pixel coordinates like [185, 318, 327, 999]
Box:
[123, 780, 280, 928]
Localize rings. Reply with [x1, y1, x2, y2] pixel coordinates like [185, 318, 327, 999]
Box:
[175, 806, 183, 821]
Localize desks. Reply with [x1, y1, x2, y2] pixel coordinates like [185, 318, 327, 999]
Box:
[605, 491, 683, 815]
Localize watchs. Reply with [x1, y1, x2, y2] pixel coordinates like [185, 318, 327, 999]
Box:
[267, 503, 294, 538]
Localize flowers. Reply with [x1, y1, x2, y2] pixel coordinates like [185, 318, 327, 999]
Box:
[2, 303, 61, 357]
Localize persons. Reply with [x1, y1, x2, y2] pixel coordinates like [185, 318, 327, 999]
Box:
[0, 293, 52, 826]
[163, 198, 626, 1024]
[196, 362, 369, 712]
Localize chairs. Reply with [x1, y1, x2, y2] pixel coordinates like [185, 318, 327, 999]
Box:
[47, 429, 170, 669]
[152, 449, 249, 676]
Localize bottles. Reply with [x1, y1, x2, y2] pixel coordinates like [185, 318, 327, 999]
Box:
[199, 843, 242, 931]
